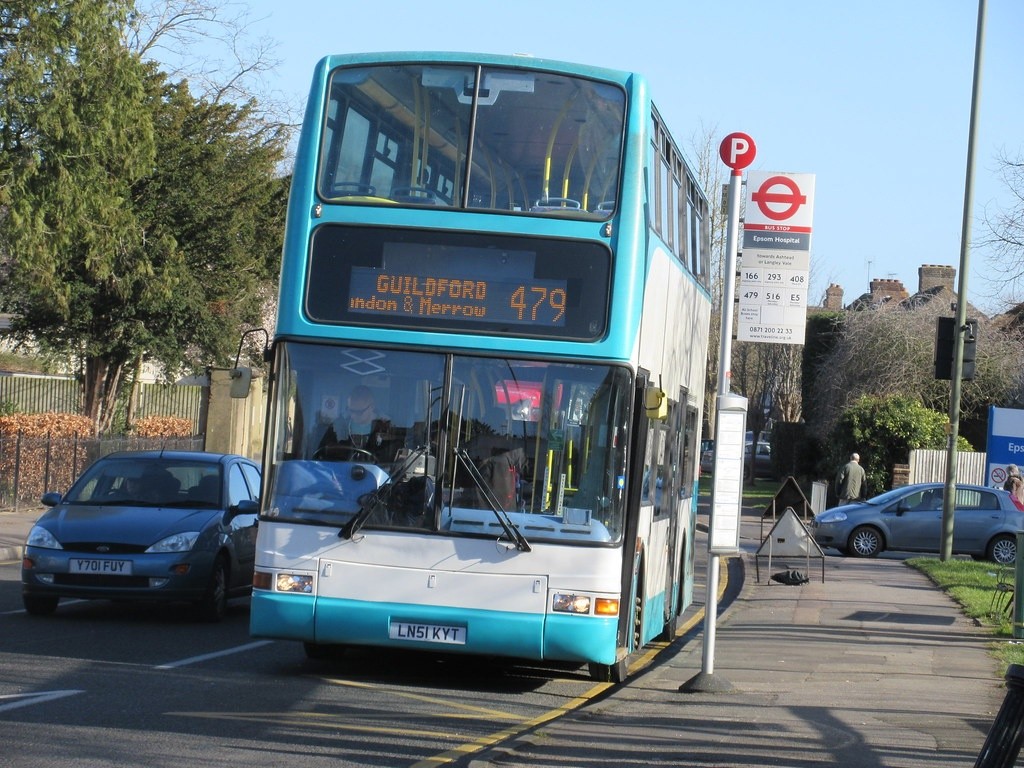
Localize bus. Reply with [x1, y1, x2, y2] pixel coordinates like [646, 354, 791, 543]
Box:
[230, 49, 718, 684]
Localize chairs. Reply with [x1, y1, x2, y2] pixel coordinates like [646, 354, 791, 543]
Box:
[388, 187, 437, 205]
[530, 197, 584, 212]
[328, 183, 377, 198]
[594, 201, 616, 213]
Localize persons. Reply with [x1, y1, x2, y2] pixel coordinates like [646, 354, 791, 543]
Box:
[835, 453, 866, 506]
[458, 408, 528, 512]
[1003, 464, 1024, 505]
[313, 385, 392, 471]
[126, 471, 143, 495]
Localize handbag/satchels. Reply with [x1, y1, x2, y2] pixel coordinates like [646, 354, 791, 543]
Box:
[357, 476, 445, 525]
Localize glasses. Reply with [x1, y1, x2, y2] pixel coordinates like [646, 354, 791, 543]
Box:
[347, 403, 371, 417]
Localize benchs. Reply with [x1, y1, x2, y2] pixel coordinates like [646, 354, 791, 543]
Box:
[989, 564, 1016, 620]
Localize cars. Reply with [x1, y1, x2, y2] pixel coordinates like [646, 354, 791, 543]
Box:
[698, 429, 773, 482]
[809, 481, 1024, 567]
[20, 449, 262, 626]
